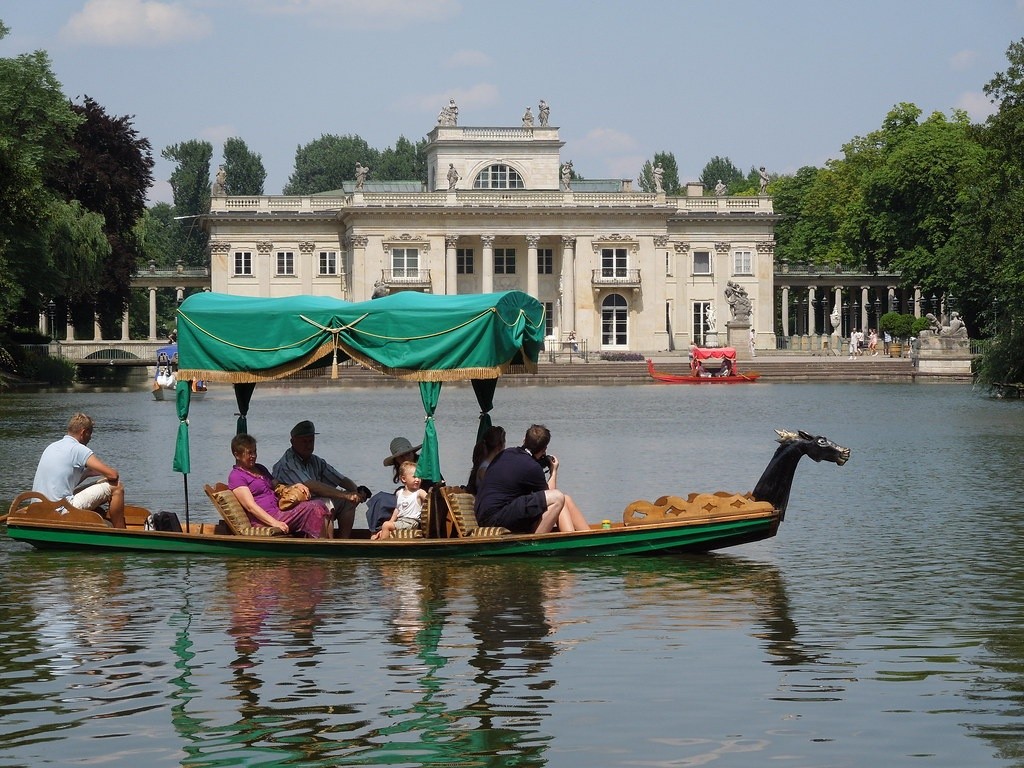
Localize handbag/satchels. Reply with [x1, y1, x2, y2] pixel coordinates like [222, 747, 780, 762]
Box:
[144, 514, 157, 532]
[152, 511, 183, 532]
[274, 484, 309, 511]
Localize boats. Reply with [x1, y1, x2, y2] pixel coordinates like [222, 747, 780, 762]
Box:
[1, 289, 852, 565]
[645, 347, 762, 383]
[152, 343, 208, 403]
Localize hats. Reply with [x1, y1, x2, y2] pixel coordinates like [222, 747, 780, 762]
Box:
[383, 437, 423, 467]
[291, 421, 320, 438]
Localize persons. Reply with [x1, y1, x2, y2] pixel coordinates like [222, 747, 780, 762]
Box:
[538, 99, 550, 126]
[228, 421, 446, 539]
[750, 329, 757, 357]
[473, 424, 565, 534]
[695, 359, 731, 377]
[715, 180, 727, 196]
[849, 327, 917, 361]
[216, 166, 227, 194]
[437, 98, 458, 126]
[468, 426, 591, 532]
[168, 328, 177, 344]
[30, 415, 126, 529]
[521, 107, 535, 127]
[562, 163, 571, 191]
[758, 166, 770, 195]
[654, 163, 665, 191]
[705, 305, 717, 331]
[354, 163, 370, 190]
[569, 331, 578, 352]
[447, 163, 462, 191]
[724, 279, 749, 319]
[920, 312, 965, 338]
[688, 342, 697, 369]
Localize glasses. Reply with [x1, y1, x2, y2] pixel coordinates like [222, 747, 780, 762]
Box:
[489, 424, 504, 440]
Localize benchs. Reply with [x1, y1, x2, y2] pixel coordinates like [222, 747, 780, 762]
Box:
[440, 485, 511, 536]
[204, 483, 293, 538]
[388, 486, 433, 539]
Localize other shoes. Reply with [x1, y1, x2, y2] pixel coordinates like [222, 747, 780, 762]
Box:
[849, 350, 890, 360]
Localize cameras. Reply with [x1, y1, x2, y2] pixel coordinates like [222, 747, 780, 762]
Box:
[537, 451, 553, 468]
